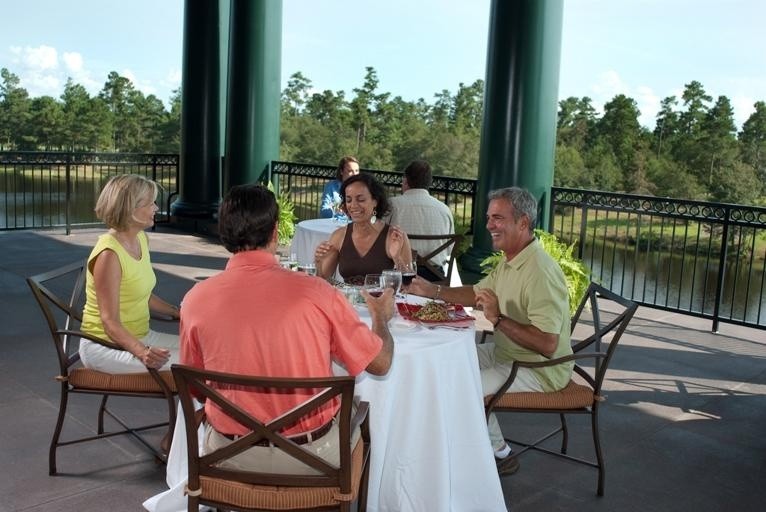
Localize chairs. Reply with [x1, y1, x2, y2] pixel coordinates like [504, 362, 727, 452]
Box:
[485, 279, 639, 497]
[170, 363, 371, 512]
[388, 233, 460, 290]
[26, 257, 188, 476]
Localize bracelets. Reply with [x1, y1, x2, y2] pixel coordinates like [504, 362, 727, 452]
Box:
[434, 284, 442, 299]
[493, 313, 504, 331]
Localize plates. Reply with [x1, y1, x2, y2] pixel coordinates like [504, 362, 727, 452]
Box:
[395, 301, 476, 324]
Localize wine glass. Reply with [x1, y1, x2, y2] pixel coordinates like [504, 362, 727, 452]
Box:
[281, 250, 417, 310]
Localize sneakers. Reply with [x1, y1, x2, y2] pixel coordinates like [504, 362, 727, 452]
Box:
[493, 450, 521, 476]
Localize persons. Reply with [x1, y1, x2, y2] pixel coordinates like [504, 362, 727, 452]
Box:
[385, 160, 457, 283]
[314, 173, 414, 286]
[320, 157, 361, 218]
[403, 186, 572, 478]
[179, 183, 396, 479]
[77, 173, 181, 459]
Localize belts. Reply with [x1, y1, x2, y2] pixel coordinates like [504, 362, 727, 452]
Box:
[222, 420, 331, 446]
